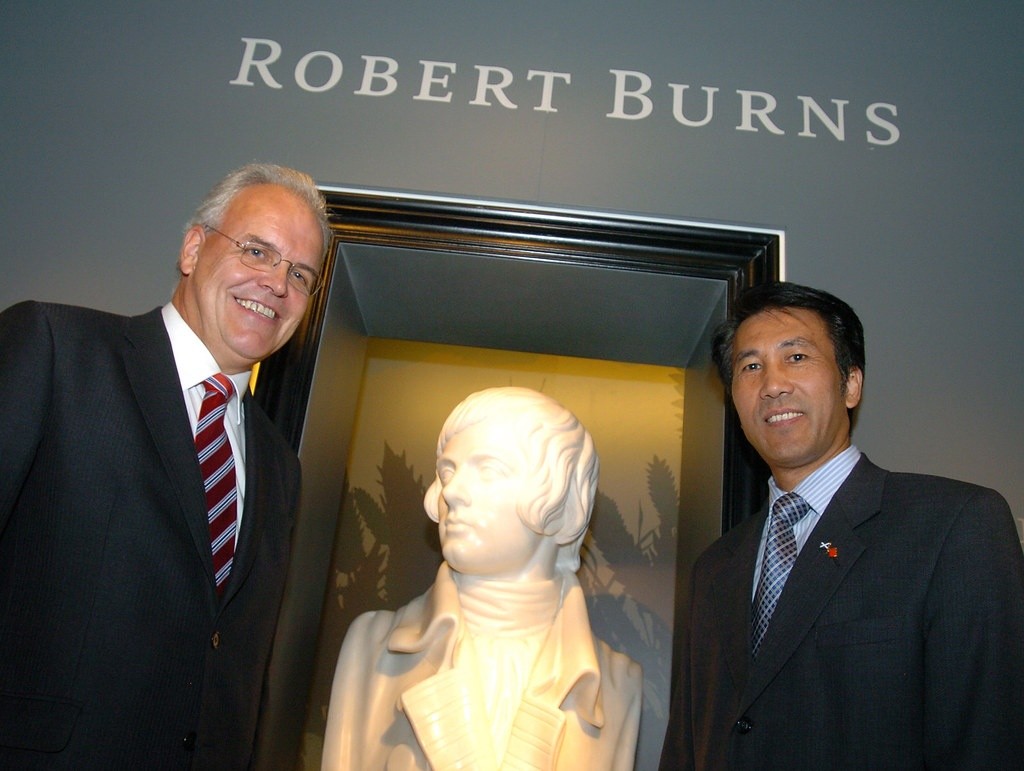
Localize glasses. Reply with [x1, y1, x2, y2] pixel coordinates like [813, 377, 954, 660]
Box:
[204, 224, 325, 297]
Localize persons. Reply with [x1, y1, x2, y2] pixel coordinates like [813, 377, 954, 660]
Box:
[321, 387, 643, 771]
[659, 281, 1024, 771]
[0, 163, 333, 771]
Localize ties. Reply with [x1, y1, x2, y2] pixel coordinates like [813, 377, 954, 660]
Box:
[751, 492, 811, 673]
[194, 373, 238, 605]
